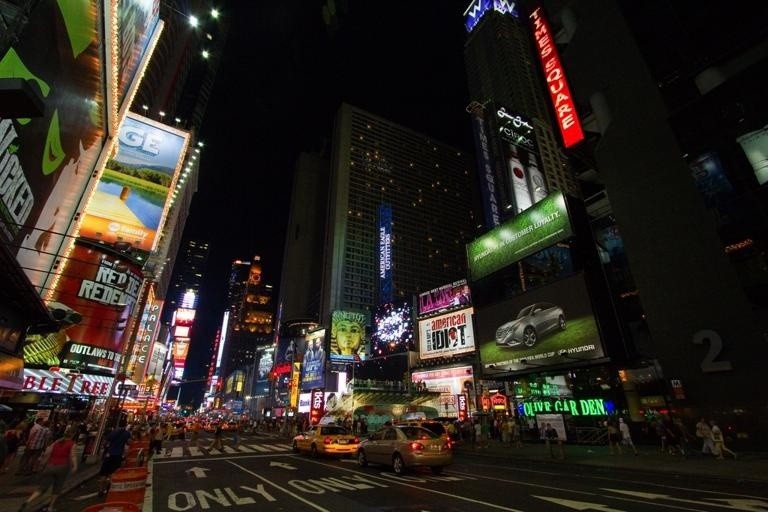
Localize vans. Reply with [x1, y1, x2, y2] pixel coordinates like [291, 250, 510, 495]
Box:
[392, 420, 453, 446]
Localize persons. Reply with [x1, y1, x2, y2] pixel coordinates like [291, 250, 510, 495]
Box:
[650, 414, 738, 461]
[332, 318, 363, 355]
[607, 420, 623, 455]
[306, 339, 314, 362]
[619, 417, 638, 456]
[314, 338, 325, 360]
[19, 425, 79, 512]
[263, 415, 368, 435]
[191, 421, 242, 451]
[453, 290, 462, 305]
[326, 393, 338, 408]
[0, 412, 174, 476]
[443, 416, 521, 449]
[539, 422, 546, 438]
[546, 423, 558, 438]
[459, 285, 471, 305]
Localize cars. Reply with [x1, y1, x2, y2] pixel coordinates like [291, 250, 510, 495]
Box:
[172, 416, 239, 439]
[496, 303, 567, 349]
[359, 426, 451, 476]
[292, 424, 360, 458]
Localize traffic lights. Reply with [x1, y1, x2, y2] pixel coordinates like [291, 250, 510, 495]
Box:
[217, 376, 224, 391]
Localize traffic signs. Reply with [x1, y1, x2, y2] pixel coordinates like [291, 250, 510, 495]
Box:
[119, 384, 136, 390]
